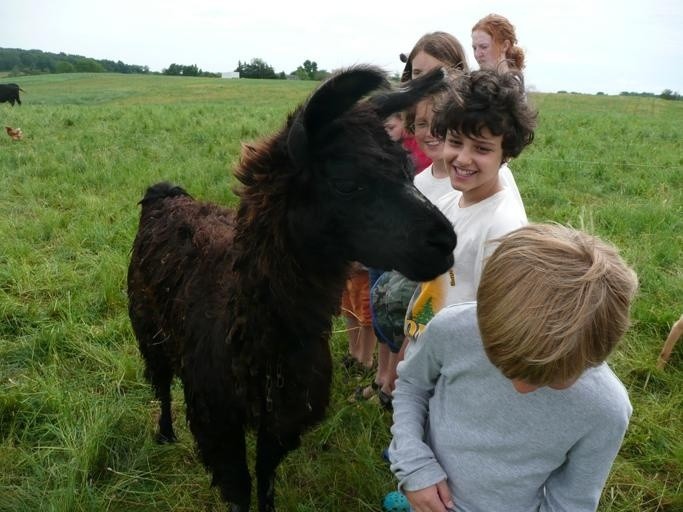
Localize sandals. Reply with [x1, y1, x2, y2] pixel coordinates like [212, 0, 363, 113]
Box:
[340, 349, 393, 412]
[383, 490, 411, 512]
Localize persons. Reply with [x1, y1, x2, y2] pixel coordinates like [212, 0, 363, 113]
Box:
[385, 58, 541, 511]
[387, 221, 639, 511]
[471, 15, 527, 101]
[336, 30, 472, 413]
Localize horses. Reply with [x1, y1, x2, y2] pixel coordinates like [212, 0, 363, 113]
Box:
[126, 62, 460, 512]
